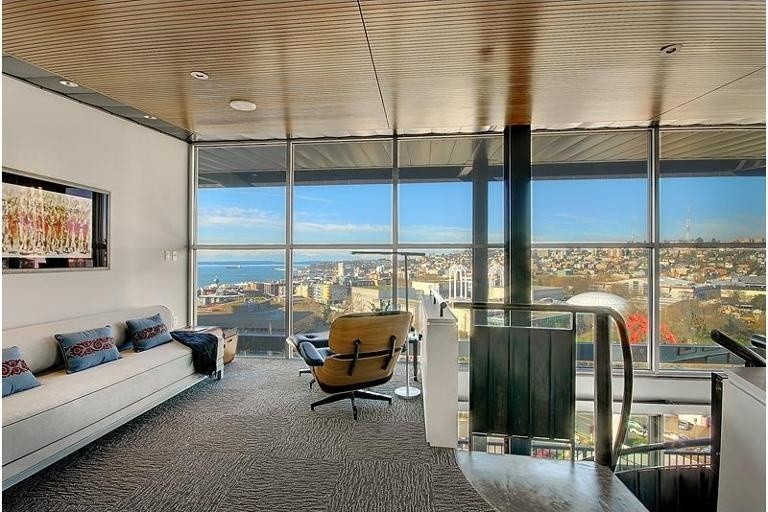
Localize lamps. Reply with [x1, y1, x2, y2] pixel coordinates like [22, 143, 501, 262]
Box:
[351, 250, 425, 400]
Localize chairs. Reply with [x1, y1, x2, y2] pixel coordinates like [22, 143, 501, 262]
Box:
[286, 310, 414, 420]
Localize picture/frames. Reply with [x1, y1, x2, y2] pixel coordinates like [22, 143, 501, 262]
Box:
[2, 166, 111, 273]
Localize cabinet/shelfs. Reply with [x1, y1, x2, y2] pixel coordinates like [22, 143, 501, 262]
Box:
[715, 367, 766, 512]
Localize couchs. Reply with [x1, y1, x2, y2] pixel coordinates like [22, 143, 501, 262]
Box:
[2, 304, 224, 495]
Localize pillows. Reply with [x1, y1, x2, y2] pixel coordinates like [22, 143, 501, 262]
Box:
[2, 346, 41, 397]
[125, 313, 174, 353]
[54, 325, 123, 374]
